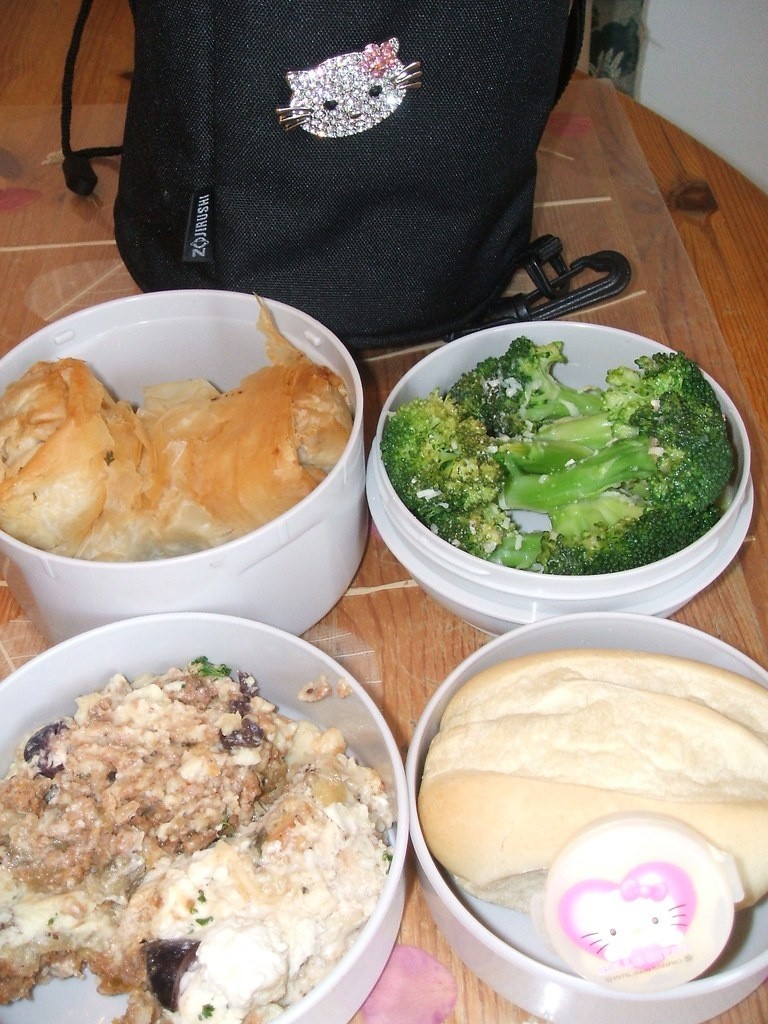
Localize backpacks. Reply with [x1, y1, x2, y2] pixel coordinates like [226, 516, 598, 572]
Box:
[61, 0, 632, 352]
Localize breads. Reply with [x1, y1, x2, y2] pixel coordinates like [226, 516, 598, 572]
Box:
[418, 647, 768, 907]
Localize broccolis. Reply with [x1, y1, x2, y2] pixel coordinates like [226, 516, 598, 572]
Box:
[382, 339, 735, 577]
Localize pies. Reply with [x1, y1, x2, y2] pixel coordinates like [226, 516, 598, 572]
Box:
[1, 357, 353, 549]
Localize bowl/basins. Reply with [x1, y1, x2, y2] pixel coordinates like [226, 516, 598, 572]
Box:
[0, 614, 408, 1024]
[0, 289, 370, 638]
[407, 612, 768, 1024]
[365, 320, 754, 637]
